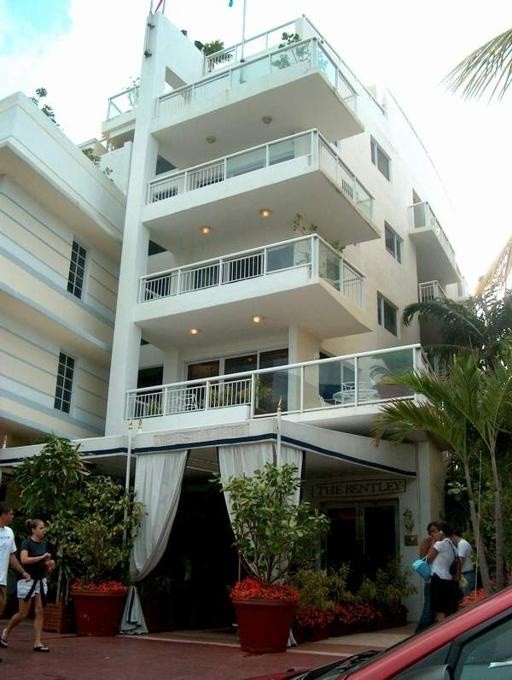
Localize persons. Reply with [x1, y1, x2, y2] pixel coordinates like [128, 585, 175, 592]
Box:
[0, 518, 57, 652]
[415, 523, 452, 634]
[0, 499, 31, 616]
[449, 527, 480, 595]
[425, 521, 462, 613]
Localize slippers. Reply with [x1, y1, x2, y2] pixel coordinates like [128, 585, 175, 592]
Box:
[32, 644, 50, 653]
[0, 634, 8, 648]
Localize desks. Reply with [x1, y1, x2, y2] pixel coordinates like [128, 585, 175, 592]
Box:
[332, 387, 377, 403]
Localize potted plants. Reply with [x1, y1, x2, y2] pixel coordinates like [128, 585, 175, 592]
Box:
[369, 345, 412, 397]
[291, 558, 417, 645]
[209, 462, 331, 652]
[43, 477, 147, 638]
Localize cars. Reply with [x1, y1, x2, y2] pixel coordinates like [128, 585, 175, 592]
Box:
[242, 584, 512, 680]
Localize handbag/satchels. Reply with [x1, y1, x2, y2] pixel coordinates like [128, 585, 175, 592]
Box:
[411, 556, 431, 580]
[449, 560, 457, 575]
